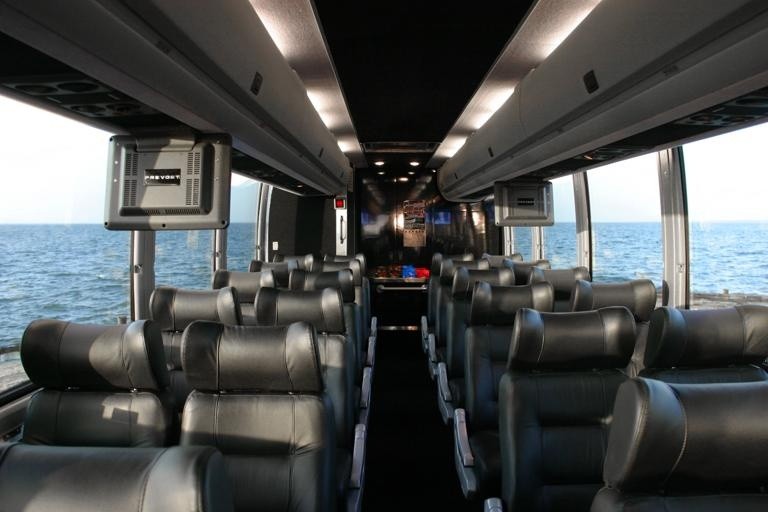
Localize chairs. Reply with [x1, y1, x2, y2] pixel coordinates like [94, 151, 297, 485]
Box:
[420, 251, 766, 510]
[1, 251, 381, 511]
